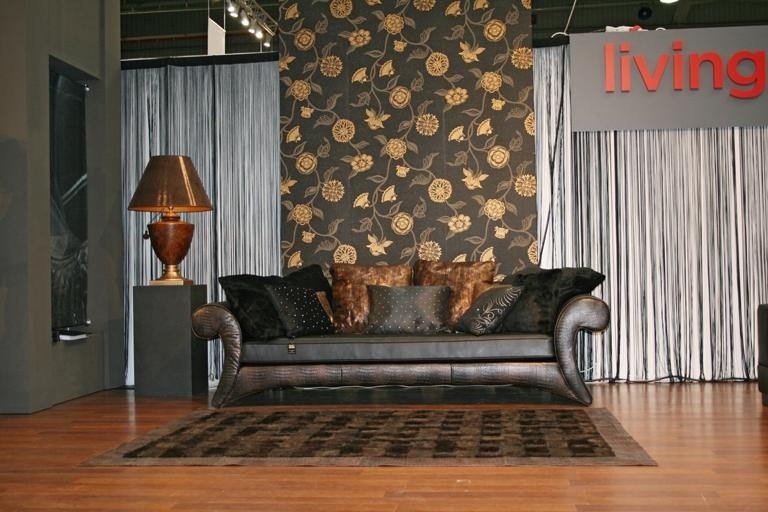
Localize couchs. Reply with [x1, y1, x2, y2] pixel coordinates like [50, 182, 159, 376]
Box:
[191, 283, 612, 409]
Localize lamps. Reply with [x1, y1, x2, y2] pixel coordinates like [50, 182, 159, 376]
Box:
[227, 0, 281, 47]
[126, 155, 214, 287]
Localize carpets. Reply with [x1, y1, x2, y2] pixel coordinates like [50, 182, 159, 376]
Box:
[78, 406, 659, 467]
